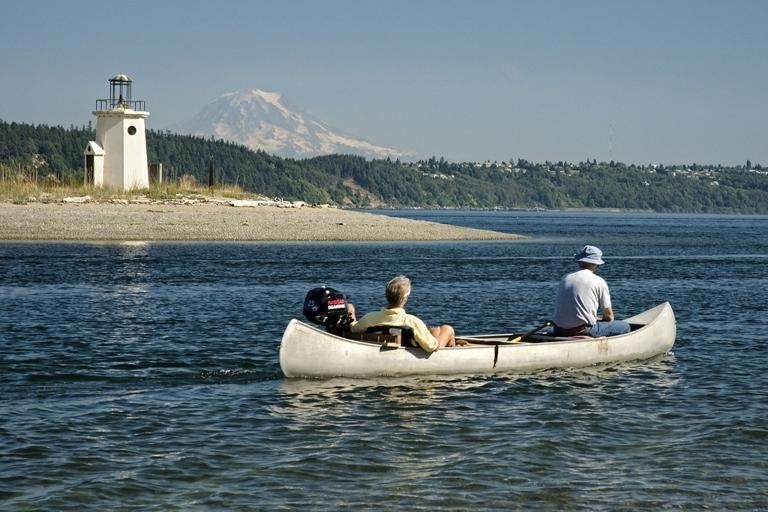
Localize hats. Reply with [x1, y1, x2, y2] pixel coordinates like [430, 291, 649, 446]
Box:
[575, 245, 605, 265]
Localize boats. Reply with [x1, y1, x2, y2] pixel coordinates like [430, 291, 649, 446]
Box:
[276, 300, 679, 376]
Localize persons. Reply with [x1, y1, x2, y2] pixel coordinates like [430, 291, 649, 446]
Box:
[342, 274, 456, 355]
[552, 244, 631, 338]
